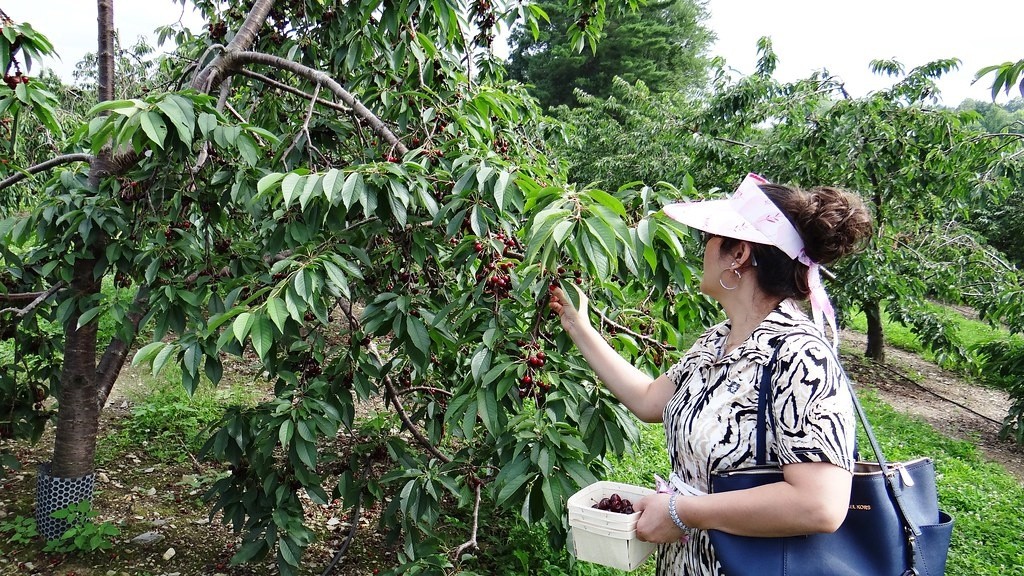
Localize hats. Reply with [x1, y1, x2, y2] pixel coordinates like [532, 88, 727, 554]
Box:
[662, 173, 837, 355]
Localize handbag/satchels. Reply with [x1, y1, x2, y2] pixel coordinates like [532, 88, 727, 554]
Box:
[708, 332, 955, 575]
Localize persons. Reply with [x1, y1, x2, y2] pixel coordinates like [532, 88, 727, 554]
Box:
[549, 172, 873, 576]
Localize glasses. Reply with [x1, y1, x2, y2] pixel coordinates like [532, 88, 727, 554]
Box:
[697, 229, 757, 266]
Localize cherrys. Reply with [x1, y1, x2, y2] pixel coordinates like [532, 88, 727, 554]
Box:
[589, 494, 634, 514]
[130, 96, 669, 401]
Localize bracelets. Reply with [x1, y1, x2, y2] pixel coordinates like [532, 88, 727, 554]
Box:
[669, 496, 690, 534]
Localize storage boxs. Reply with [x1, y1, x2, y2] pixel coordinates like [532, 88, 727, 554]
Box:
[566, 480, 658, 573]
